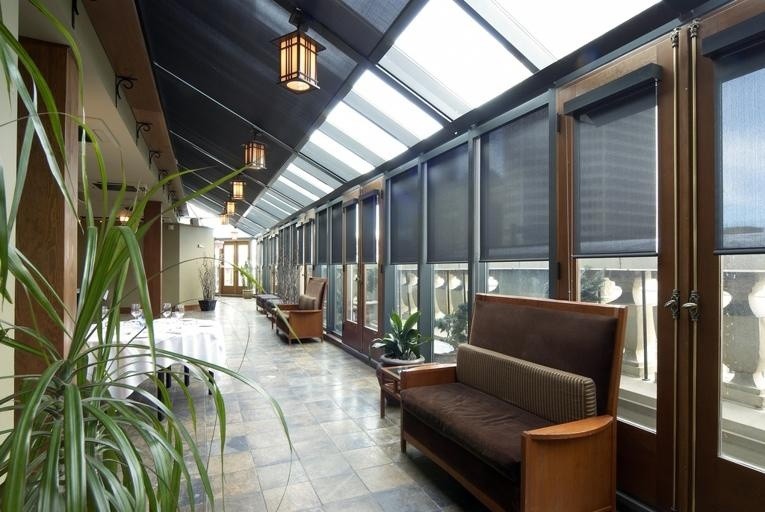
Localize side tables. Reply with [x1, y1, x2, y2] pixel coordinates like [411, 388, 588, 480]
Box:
[380, 363, 441, 419]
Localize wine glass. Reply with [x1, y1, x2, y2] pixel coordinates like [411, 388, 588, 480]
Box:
[130, 303, 185, 323]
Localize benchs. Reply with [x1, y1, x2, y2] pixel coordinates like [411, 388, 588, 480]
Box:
[276, 277, 328, 345]
[399, 291, 630, 512]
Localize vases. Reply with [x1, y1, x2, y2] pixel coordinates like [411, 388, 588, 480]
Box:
[198, 299, 217, 311]
[242, 289, 253, 299]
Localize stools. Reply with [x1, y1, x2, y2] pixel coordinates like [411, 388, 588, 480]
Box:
[256, 294, 285, 320]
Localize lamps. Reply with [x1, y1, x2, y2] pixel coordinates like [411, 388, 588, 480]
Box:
[218, 123, 268, 226]
[269, 9, 327, 96]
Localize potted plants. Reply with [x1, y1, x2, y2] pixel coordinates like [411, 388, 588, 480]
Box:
[370, 310, 435, 389]
